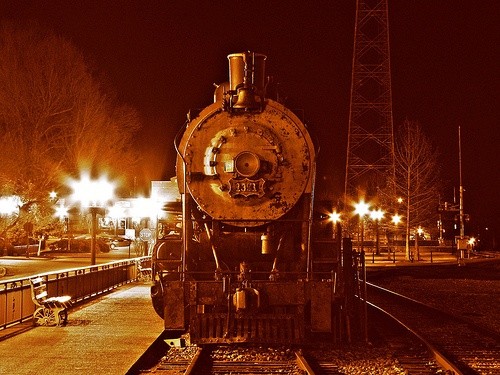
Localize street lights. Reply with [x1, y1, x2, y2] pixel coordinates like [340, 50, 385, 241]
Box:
[79, 178, 110, 266]
[369, 208, 384, 257]
[356, 199, 375, 255]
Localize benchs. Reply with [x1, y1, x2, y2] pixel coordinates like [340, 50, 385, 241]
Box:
[136, 261, 152, 282]
[30, 277, 71, 327]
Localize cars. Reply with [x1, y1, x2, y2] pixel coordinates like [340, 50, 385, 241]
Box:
[108, 238, 130, 248]
[8, 235, 40, 255]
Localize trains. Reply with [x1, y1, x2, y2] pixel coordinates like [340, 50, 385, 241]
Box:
[148, 51, 358, 348]
